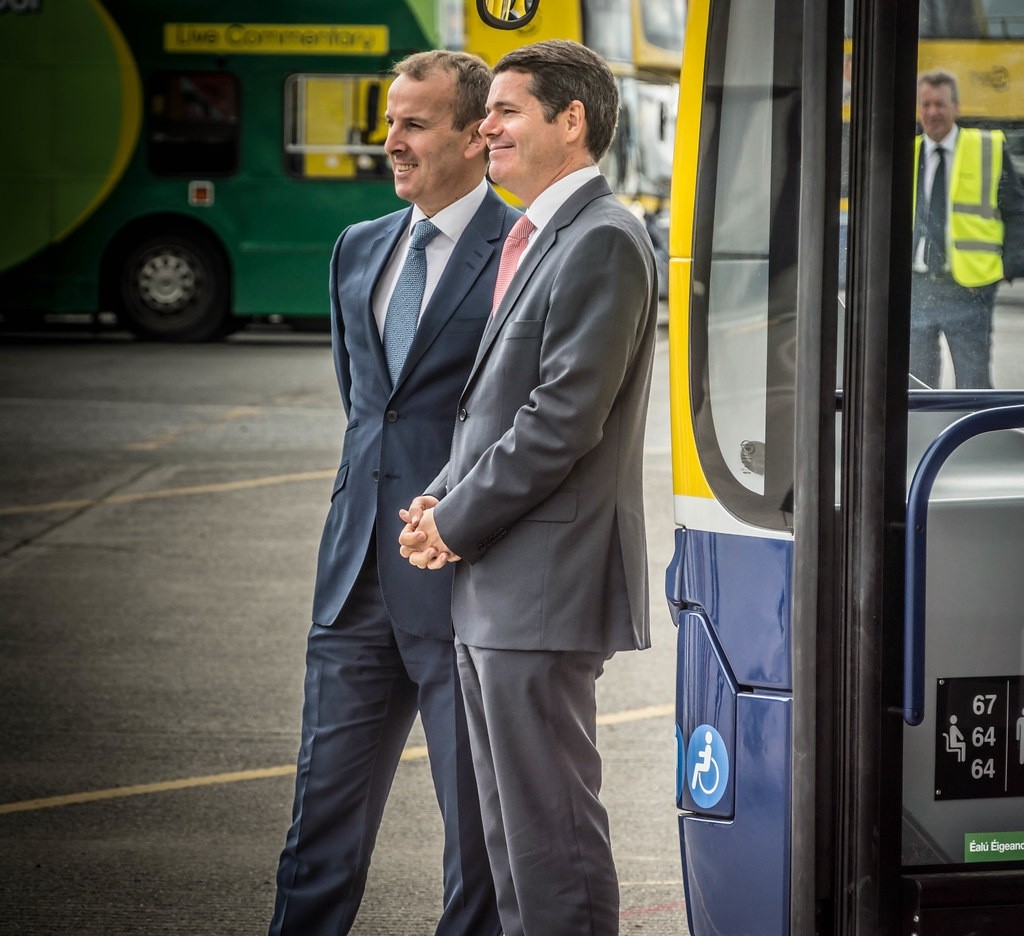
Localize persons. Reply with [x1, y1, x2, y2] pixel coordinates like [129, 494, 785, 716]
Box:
[399, 40, 657, 936]
[267, 51, 503, 936]
[909, 70, 1024, 390]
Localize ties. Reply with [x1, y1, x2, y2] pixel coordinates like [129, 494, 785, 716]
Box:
[922, 147, 947, 276]
[491, 215, 535, 318]
[383, 219, 442, 389]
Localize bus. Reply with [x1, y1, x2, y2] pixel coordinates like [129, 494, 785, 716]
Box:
[1, 0, 464, 342]
[447, 0, 1024, 307]
[663, 0, 1024, 936]
